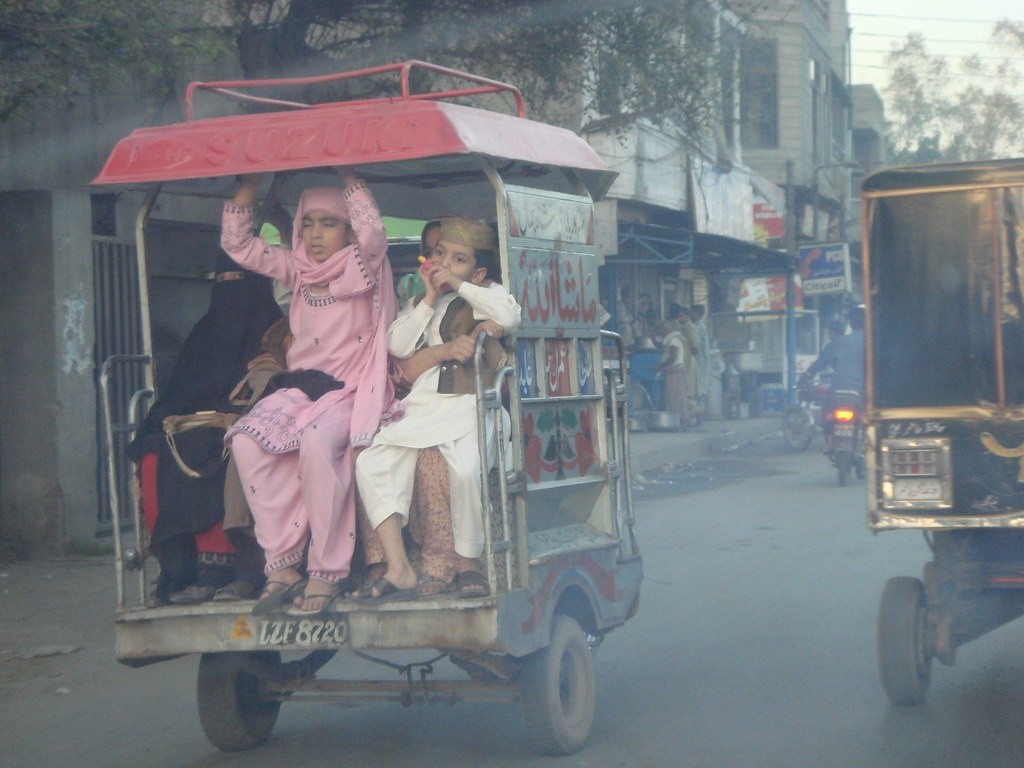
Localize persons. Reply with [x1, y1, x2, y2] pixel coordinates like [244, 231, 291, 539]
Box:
[345, 216, 521, 602]
[797, 307, 867, 478]
[219, 164, 405, 615]
[127, 203, 294, 604]
[648, 304, 707, 425]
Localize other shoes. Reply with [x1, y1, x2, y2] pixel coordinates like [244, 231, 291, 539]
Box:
[213, 579, 258, 601]
[170, 584, 215, 604]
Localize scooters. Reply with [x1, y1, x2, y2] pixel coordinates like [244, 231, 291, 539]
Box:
[783, 374, 865, 485]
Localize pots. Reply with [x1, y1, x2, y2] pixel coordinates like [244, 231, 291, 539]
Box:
[730, 353, 762, 372]
[646, 412, 679, 430]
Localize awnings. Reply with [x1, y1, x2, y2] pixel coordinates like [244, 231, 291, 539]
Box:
[619, 221, 800, 271]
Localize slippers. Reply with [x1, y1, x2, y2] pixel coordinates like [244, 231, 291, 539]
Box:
[458, 571, 488, 598]
[348, 576, 417, 605]
[252, 578, 309, 615]
[287, 587, 342, 615]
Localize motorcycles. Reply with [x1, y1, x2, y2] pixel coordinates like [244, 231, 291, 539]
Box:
[87, 58, 642, 756]
[864, 156, 1024, 704]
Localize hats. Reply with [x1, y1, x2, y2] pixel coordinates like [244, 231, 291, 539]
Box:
[438, 217, 494, 251]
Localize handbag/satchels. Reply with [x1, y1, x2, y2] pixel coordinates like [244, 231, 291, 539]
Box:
[162, 413, 243, 477]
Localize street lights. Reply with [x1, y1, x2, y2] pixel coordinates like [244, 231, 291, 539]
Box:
[813, 161, 860, 353]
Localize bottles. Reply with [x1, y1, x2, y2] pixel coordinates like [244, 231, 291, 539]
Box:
[418, 255, 452, 292]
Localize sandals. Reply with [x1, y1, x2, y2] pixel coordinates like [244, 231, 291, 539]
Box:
[416, 576, 458, 596]
